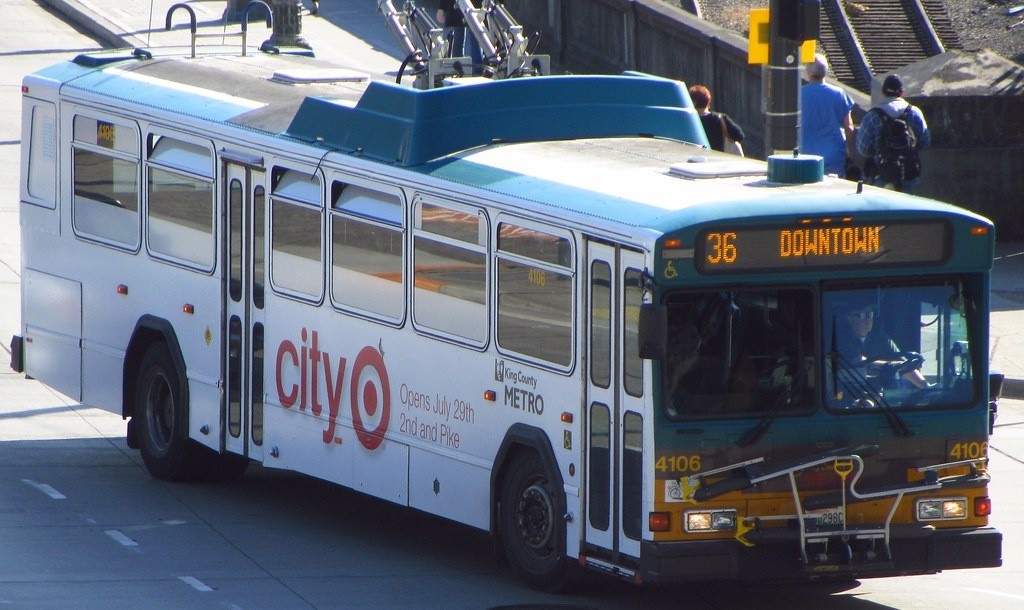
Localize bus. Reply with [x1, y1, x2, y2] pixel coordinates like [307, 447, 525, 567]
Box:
[9, 0, 1006, 595]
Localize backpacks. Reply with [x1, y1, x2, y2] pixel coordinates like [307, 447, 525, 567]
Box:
[867, 104, 922, 183]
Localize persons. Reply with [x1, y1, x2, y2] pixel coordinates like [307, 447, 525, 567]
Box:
[437, 0, 466, 58]
[800, 52, 856, 178]
[856, 74, 931, 195]
[834, 298, 944, 392]
[688, 84, 745, 154]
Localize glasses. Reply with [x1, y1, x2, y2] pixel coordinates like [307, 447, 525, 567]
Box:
[847, 311, 874, 320]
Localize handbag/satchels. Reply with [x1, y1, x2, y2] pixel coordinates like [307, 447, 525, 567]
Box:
[719, 113, 745, 157]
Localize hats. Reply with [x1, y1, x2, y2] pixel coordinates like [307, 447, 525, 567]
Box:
[805, 53, 828, 76]
[883, 74, 903, 94]
[849, 300, 878, 313]
